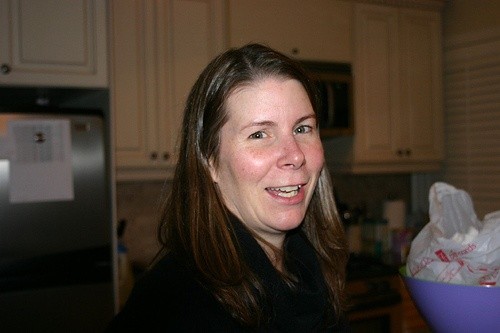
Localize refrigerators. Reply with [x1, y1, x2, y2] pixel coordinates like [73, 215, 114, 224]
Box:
[0, 86, 114, 333]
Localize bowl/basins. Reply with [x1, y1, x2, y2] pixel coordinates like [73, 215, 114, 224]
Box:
[398, 267, 500, 333]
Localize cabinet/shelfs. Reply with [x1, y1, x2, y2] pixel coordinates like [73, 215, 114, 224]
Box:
[232, 0, 357, 64]
[109, 0, 233, 171]
[0, 0, 109, 90]
[322, 2, 448, 175]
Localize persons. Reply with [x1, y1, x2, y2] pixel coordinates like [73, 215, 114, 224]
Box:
[106, 43, 346, 333]
